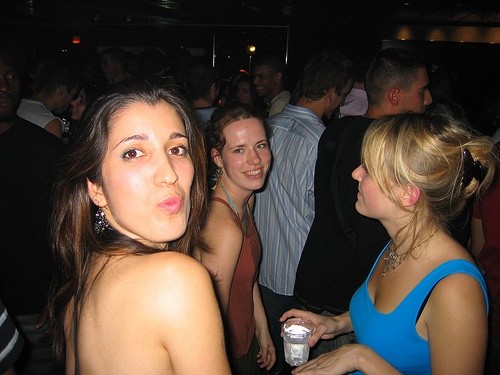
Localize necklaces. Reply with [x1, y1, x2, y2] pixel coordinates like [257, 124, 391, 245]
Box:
[381, 226, 440, 276]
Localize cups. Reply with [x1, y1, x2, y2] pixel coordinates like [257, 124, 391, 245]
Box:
[281, 320, 315, 365]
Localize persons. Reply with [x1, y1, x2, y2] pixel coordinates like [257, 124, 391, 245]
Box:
[0, 48, 127, 375]
[235, 52, 354, 375]
[445, 141, 500, 375]
[279, 112, 495, 375]
[192, 102, 276, 375]
[50, 86, 230, 375]
[170, 47, 368, 128]
[293, 48, 433, 359]
